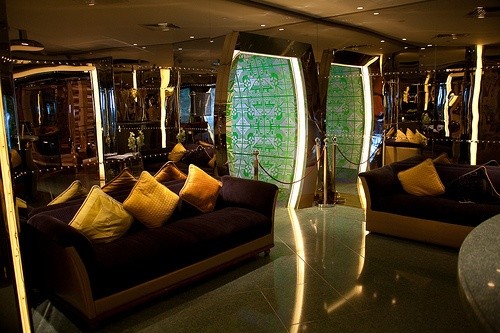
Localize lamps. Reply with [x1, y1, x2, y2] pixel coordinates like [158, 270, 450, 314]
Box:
[448, 91, 458, 106]
[10, 29, 46, 52]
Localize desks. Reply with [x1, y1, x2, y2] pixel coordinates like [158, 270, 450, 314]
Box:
[6, 135, 39, 150]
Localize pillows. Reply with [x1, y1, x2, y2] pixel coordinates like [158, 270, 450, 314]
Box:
[101, 166, 139, 193]
[167, 141, 187, 163]
[121, 171, 180, 230]
[451, 165, 500, 202]
[432, 153, 451, 165]
[406, 128, 419, 144]
[179, 163, 224, 214]
[67, 185, 134, 244]
[395, 129, 409, 142]
[414, 129, 427, 145]
[46, 180, 90, 206]
[153, 161, 188, 183]
[398, 158, 446, 197]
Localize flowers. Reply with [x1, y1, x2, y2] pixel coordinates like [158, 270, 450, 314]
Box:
[135, 129, 145, 156]
[175, 127, 186, 143]
[127, 131, 137, 160]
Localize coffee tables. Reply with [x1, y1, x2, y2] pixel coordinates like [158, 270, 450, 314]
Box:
[458, 214, 500, 333]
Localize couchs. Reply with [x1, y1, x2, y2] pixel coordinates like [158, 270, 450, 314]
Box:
[30, 126, 61, 155]
[167, 141, 215, 174]
[378, 138, 432, 168]
[356, 161, 500, 249]
[24, 173, 279, 333]
